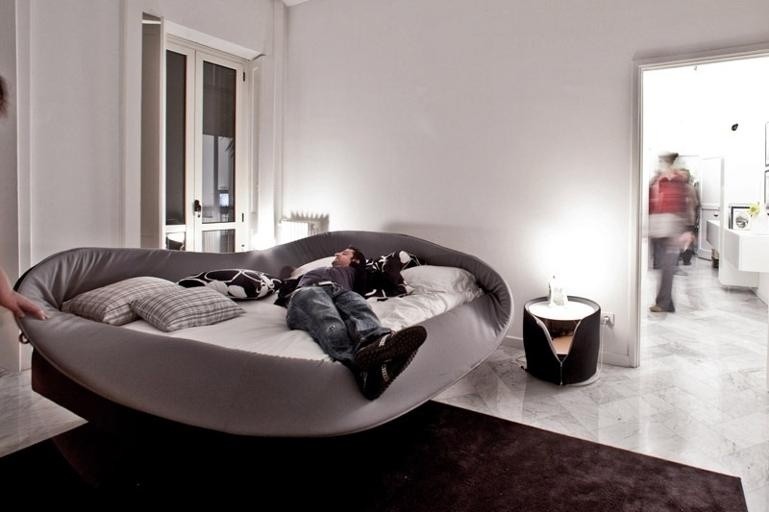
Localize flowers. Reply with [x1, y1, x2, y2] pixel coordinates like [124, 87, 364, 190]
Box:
[746, 203, 765, 216]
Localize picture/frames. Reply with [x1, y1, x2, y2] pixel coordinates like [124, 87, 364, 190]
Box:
[731, 206, 752, 231]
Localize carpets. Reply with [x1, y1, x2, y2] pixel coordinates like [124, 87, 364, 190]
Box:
[0, 400, 748, 512]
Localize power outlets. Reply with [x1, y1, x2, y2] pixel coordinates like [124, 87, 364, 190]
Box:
[600, 311, 614, 325]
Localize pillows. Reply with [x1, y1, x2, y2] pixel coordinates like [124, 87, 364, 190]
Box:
[127, 286, 247, 333]
[58, 275, 186, 327]
[175, 268, 281, 300]
[287, 254, 368, 280]
[400, 264, 478, 294]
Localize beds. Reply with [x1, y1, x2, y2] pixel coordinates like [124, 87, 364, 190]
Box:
[12, 230, 514, 437]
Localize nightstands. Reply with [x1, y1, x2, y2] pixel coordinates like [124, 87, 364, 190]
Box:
[523, 296, 601, 387]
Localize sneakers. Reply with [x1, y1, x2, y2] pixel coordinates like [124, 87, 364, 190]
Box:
[650, 304, 675, 314]
[352, 326, 427, 400]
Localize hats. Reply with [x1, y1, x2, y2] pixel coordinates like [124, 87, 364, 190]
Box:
[659, 150, 678, 163]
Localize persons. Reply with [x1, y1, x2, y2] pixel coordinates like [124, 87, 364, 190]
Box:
[277, 244, 429, 400]
[0, 267, 53, 321]
[646, 150, 702, 313]
